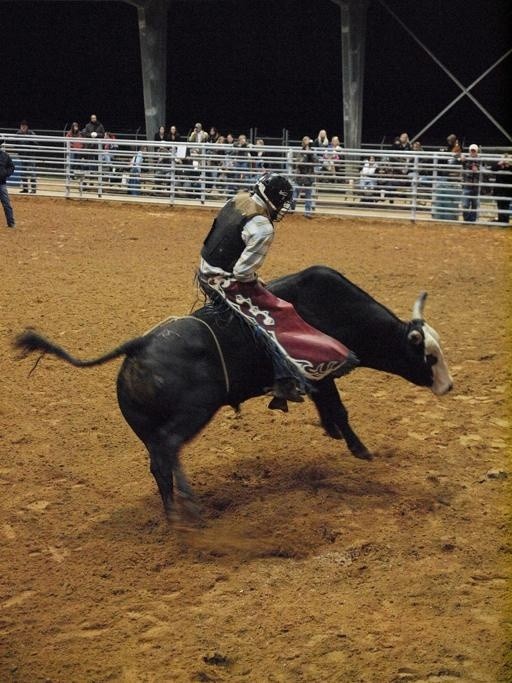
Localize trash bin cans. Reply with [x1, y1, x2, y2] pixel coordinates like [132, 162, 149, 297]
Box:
[431, 183, 462, 220]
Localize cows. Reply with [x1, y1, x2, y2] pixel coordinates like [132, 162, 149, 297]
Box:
[7, 267, 454, 522]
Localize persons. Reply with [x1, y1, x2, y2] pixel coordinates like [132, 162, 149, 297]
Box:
[195, 169, 307, 404]
[288, 130, 342, 219]
[129, 123, 268, 195]
[67, 115, 116, 178]
[6, 119, 42, 194]
[359, 134, 426, 189]
[439, 135, 511, 224]
[0, 134, 16, 228]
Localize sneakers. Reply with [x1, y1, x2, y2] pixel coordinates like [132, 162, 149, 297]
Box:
[269, 397, 288, 413]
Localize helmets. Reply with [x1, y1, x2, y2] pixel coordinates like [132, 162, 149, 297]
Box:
[250, 172, 293, 222]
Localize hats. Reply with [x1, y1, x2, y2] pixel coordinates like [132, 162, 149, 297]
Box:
[469, 144, 478, 154]
